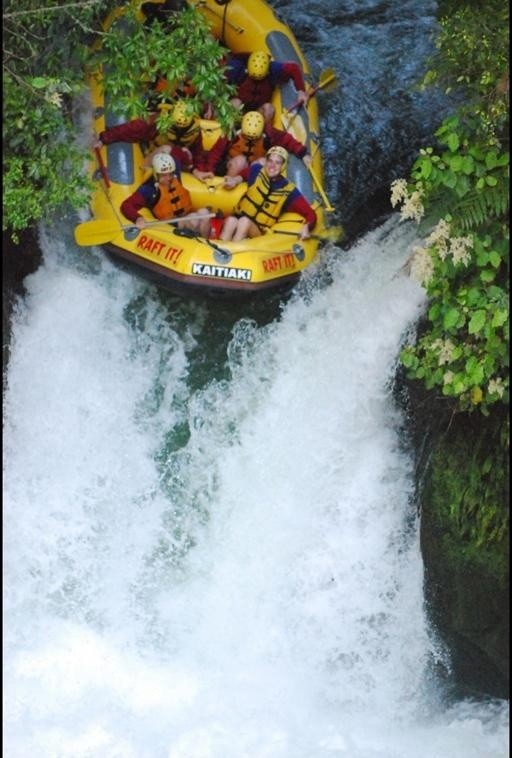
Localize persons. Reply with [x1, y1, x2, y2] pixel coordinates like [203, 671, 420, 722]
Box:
[94, 50, 312, 176]
[219, 146, 317, 240]
[120, 153, 211, 239]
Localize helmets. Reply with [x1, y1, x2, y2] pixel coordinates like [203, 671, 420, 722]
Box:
[152, 153, 177, 182]
[241, 110, 265, 141]
[264, 145, 290, 173]
[172, 102, 194, 127]
[247, 49, 270, 81]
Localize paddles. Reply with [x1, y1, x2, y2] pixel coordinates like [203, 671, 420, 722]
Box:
[74, 213, 218, 246]
[280, 113, 335, 212]
[284, 68, 341, 117]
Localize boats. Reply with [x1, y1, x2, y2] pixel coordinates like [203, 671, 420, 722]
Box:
[81, 0, 324, 299]
